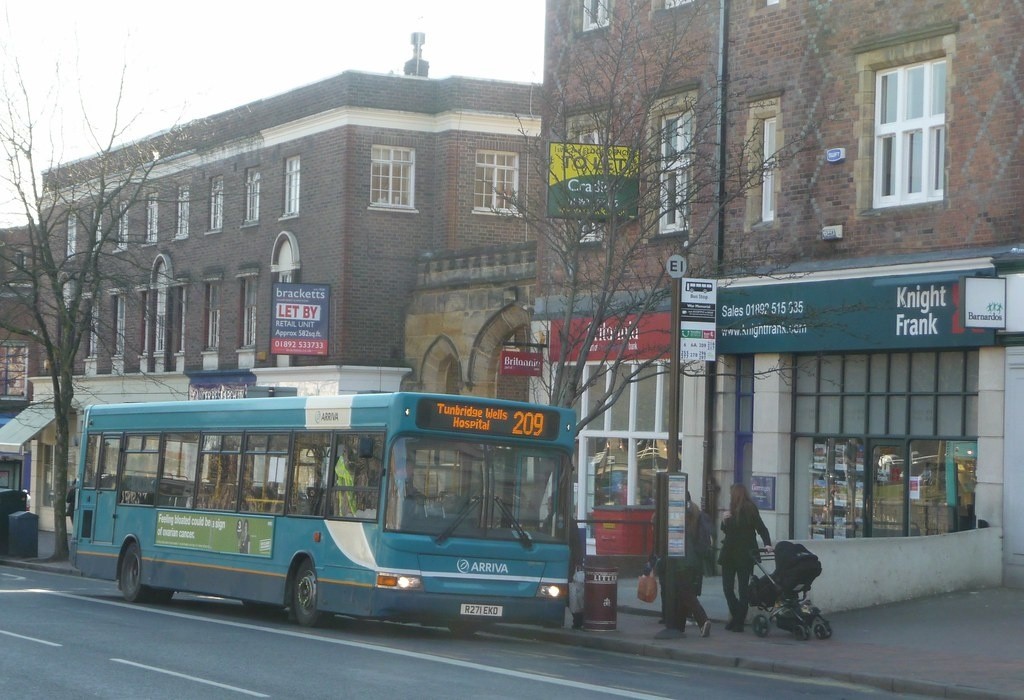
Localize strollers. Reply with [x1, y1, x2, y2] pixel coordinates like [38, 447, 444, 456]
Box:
[747, 541, 833, 641]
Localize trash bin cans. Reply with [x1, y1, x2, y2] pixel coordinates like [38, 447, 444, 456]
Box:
[591, 503, 657, 556]
[581, 567, 619, 633]
[0, 488, 29, 556]
[8, 510, 39, 557]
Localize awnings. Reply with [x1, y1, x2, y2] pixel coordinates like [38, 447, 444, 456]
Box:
[0, 403, 56, 453]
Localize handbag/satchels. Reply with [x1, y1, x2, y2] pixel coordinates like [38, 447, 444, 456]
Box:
[637, 574, 657, 603]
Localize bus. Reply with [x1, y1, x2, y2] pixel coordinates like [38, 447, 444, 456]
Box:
[70, 390, 579, 637]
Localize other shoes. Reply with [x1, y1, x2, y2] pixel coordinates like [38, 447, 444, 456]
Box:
[701, 620, 710, 637]
[726, 621, 744, 632]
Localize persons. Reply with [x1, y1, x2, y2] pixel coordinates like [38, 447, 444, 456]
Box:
[67, 478, 76, 522]
[541, 496, 583, 628]
[240, 520, 250, 554]
[640, 491, 711, 637]
[718, 483, 773, 633]
[397, 458, 423, 512]
